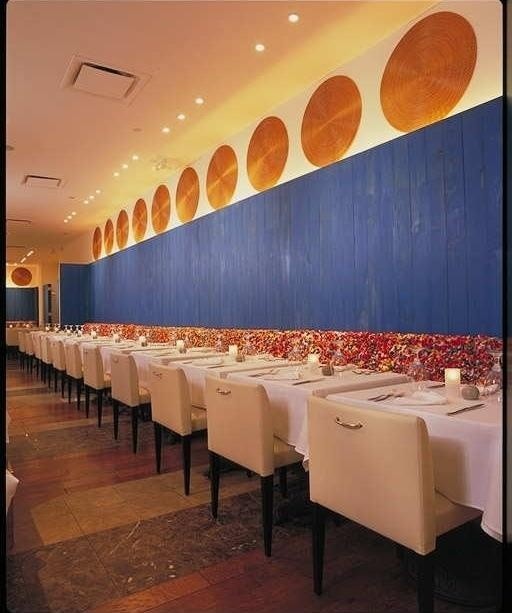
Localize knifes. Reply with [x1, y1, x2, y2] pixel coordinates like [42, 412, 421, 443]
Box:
[447, 403, 485, 416]
[368, 394, 387, 401]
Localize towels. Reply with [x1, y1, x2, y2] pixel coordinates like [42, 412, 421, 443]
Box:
[392, 389, 447, 406]
[263, 371, 300, 381]
[194, 358, 223, 366]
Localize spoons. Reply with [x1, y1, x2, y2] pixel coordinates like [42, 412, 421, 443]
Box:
[373, 392, 394, 402]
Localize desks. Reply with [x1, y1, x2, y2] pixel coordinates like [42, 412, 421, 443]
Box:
[54, 334, 177, 349]
[228, 364, 407, 472]
[327, 379, 502, 538]
[168, 354, 303, 410]
[130, 349, 226, 395]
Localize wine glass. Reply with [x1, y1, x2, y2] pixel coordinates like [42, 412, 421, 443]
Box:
[405, 345, 430, 397]
[484, 350, 502, 406]
[329, 339, 348, 383]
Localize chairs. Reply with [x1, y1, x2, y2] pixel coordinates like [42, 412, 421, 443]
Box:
[18, 331, 40, 380]
[82, 342, 111, 428]
[110, 353, 151, 453]
[64, 341, 84, 411]
[40, 335, 52, 388]
[50, 339, 66, 398]
[6, 327, 18, 360]
[204, 374, 304, 557]
[307, 395, 482, 613]
[149, 361, 207, 496]
